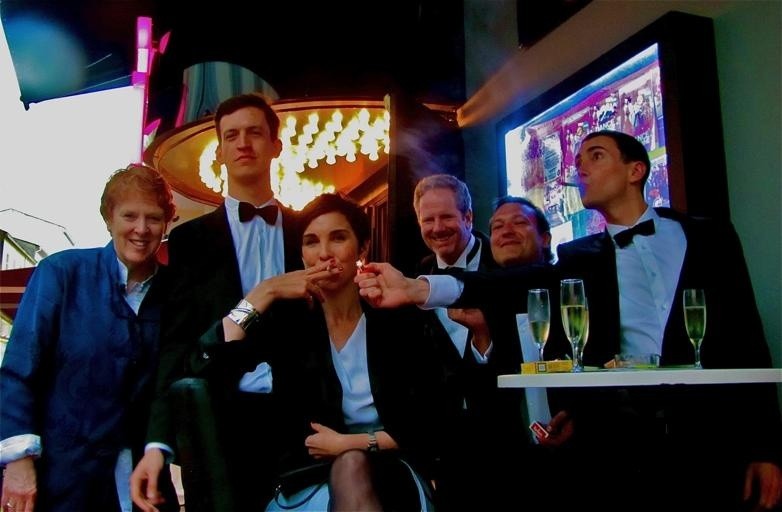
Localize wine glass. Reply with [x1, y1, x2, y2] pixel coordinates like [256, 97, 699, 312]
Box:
[527, 288, 550, 361]
[560, 279, 589, 373]
[683, 289, 706, 370]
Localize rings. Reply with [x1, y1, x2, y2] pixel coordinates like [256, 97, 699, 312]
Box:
[3, 501, 15, 512]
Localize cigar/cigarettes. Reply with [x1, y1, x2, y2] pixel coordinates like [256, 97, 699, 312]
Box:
[561, 181, 582, 189]
[324, 262, 331, 273]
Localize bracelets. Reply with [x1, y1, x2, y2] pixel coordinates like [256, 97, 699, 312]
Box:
[229, 298, 261, 333]
[366, 431, 380, 456]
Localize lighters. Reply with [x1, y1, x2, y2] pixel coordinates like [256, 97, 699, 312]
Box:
[355, 258, 367, 273]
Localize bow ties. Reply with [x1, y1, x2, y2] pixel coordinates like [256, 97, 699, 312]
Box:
[613, 218, 656, 249]
[238, 201, 279, 226]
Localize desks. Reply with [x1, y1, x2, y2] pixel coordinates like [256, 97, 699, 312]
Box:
[496, 367, 781, 389]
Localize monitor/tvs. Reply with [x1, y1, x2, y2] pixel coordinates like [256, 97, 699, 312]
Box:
[495, 11, 729, 265]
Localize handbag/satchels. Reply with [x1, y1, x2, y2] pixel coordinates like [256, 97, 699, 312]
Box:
[277, 418, 387, 497]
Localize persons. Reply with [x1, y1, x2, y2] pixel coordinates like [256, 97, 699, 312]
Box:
[352, 130, 782, 511]
[128, 89, 302, 511]
[0, 162, 181, 512]
[188, 191, 448, 512]
[403, 173, 504, 512]
[447, 194, 578, 512]
